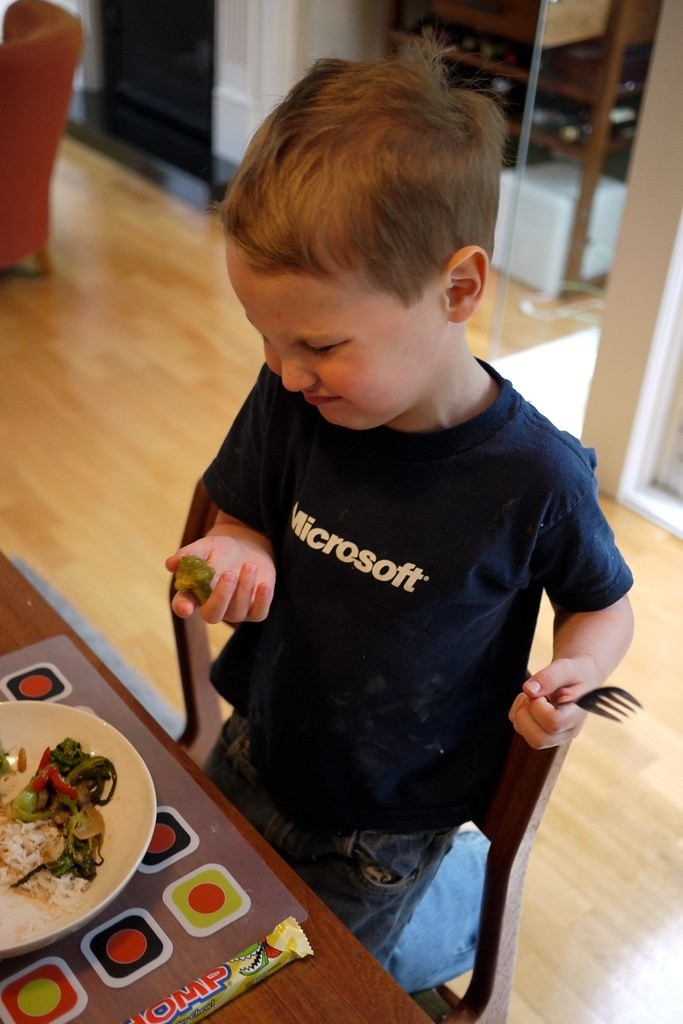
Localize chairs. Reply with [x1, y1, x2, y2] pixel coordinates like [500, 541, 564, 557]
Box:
[174, 477, 594, 1024]
[0, 0, 83, 279]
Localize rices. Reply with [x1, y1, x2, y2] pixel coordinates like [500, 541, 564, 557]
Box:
[0, 801, 90, 944]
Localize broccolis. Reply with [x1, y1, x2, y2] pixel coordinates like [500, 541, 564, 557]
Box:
[174, 555, 215, 605]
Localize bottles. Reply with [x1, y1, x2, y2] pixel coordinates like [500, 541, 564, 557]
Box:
[423, 21, 638, 140]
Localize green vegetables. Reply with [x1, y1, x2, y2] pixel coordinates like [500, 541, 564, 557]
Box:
[0, 736, 117, 889]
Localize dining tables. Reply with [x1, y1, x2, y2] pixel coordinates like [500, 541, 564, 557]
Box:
[0, 550, 444, 1024]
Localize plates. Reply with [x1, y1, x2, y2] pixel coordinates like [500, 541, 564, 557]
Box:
[0, 700, 156, 956]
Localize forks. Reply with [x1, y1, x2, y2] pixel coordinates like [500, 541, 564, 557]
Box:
[553, 687, 644, 723]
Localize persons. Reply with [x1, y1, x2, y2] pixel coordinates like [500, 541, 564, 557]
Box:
[165, 58, 633, 993]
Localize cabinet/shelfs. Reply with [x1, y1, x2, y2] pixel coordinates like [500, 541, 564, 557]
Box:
[383, 0, 665, 305]
